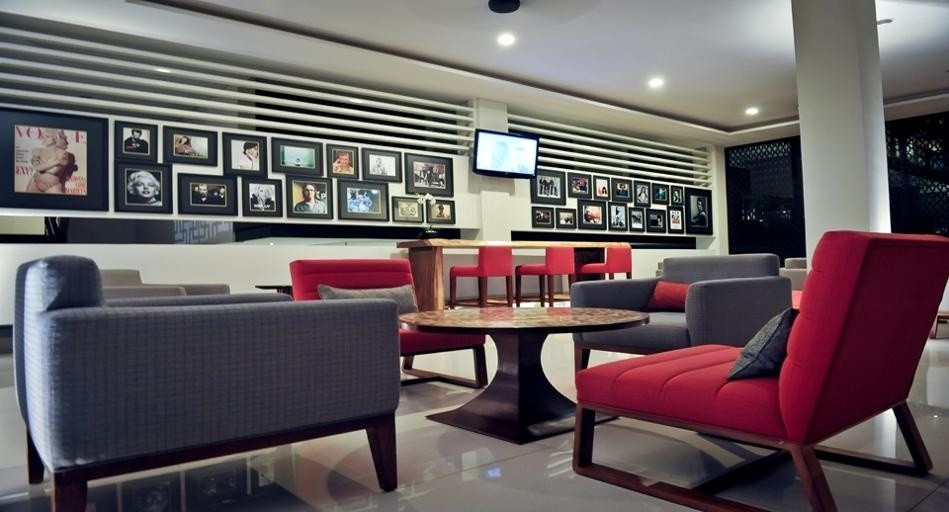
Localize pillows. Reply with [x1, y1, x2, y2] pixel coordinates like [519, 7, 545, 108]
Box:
[640, 278, 692, 313]
[727, 305, 800, 381]
[314, 281, 420, 318]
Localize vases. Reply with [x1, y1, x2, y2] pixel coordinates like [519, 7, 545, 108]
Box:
[419, 229, 441, 240]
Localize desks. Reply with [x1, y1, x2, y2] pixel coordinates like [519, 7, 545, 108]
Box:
[397, 237, 631, 313]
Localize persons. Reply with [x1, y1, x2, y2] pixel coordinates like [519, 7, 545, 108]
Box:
[249, 184, 275, 211]
[193, 184, 217, 204]
[333, 150, 354, 175]
[231, 141, 259, 171]
[295, 182, 327, 213]
[413, 162, 445, 188]
[536, 211, 549, 223]
[219, 186, 225, 200]
[672, 188, 680, 202]
[655, 215, 663, 227]
[584, 210, 600, 223]
[636, 186, 648, 204]
[292, 158, 302, 167]
[174, 134, 196, 157]
[616, 184, 628, 196]
[601, 186, 608, 197]
[124, 127, 149, 156]
[126, 171, 163, 205]
[212, 189, 219, 197]
[690, 196, 708, 227]
[436, 203, 447, 217]
[539, 176, 559, 197]
[22, 126, 77, 197]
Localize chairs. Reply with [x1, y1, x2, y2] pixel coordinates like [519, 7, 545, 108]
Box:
[287, 257, 490, 390]
[97, 266, 232, 299]
[449, 245, 513, 308]
[580, 245, 632, 280]
[12, 255, 401, 512]
[570, 229, 948, 512]
[515, 245, 576, 307]
[778, 257, 808, 290]
[570, 252, 792, 375]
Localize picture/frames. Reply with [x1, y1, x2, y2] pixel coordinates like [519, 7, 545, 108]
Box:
[530, 168, 714, 235]
[0, 104, 457, 224]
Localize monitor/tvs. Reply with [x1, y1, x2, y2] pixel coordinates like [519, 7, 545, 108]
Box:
[473, 128, 540, 179]
